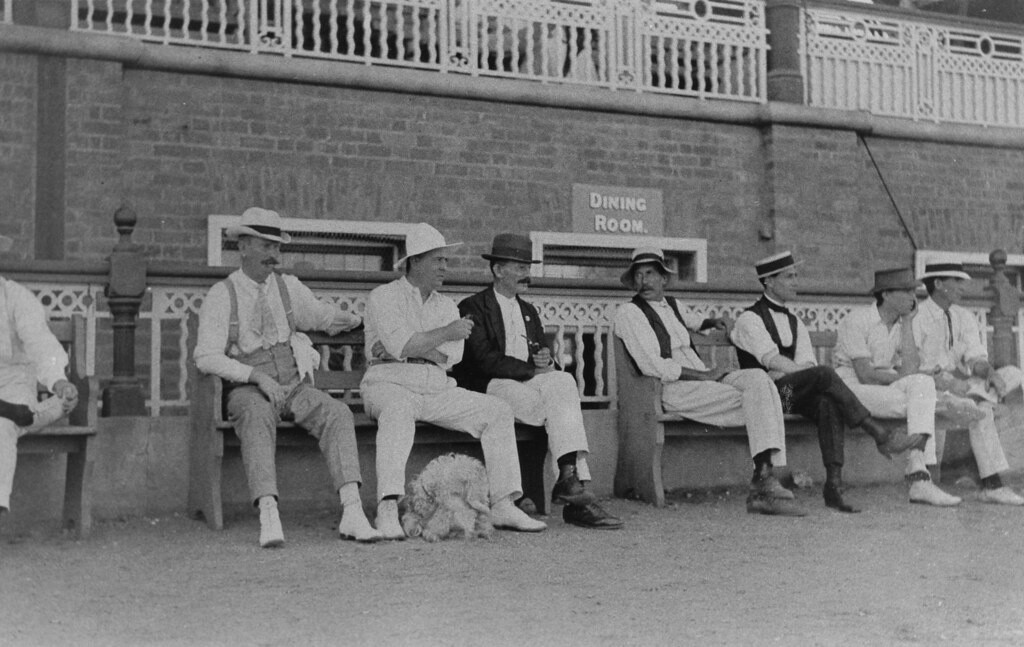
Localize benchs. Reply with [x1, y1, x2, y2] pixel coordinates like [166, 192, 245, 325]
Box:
[185, 310, 554, 530]
[611, 331, 1010, 517]
[17, 314, 100, 538]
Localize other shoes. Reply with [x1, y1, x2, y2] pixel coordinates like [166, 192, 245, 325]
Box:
[18, 391, 77, 435]
[749, 474, 794, 499]
[339, 506, 383, 541]
[877, 431, 923, 459]
[261, 507, 283, 546]
[978, 486, 1024, 506]
[489, 498, 547, 531]
[909, 480, 961, 506]
[822, 483, 861, 513]
[375, 500, 405, 539]
[745, 496, 808, 516]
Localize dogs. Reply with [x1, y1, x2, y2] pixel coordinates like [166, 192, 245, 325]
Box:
[401, 452, 494, 541]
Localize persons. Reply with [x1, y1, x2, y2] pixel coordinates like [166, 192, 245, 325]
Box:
[912, 256, 1024, 505]
[833, 267, 961, 506]
[616, 244, 807, 516]
[452, 233, 623, 525]
[731, 250, 922, 512]
[361, 221, 547, 537]
[0, 276, 78, 513]
[192, 206, 380, 546]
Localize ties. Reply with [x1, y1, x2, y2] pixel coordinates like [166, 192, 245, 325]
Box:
[946, 309, 953, 351]
[252, 284, 279, 348]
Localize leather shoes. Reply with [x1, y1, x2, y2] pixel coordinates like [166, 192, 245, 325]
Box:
[550, 474, 595, 504]
[563, 502, 624, 530]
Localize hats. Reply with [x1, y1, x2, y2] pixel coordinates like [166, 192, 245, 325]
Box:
[394, 223, 463, 268]
[917, 259, 971, 280]
[755, 251, 804, 279]
[479, 233, 541, 263]
[618, 246, 677, 290]
[225, 206, 291, 244]
[870, 267, 922, 294]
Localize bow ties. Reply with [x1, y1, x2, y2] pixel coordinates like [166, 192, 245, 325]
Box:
[762, 294, 789, 314]
